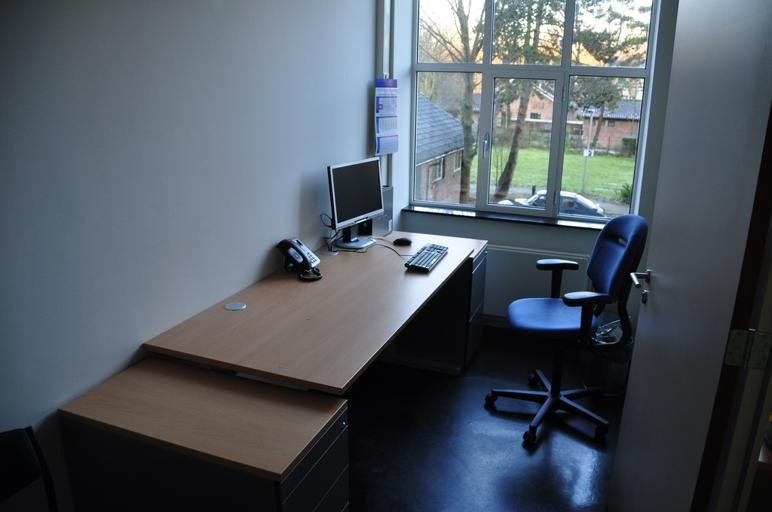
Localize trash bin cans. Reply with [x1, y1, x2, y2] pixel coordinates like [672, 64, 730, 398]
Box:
[582, 336, 633, 397]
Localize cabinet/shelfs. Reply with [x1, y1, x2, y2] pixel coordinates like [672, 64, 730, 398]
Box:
[56, 357, 353, 512]
[374, 238, 488, 378]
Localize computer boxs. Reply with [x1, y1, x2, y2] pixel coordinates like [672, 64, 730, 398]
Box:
[358, 186, 393, 240]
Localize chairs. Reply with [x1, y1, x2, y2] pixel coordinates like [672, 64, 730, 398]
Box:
[482, 213, 648, 448]
[0, 422, 59, 512]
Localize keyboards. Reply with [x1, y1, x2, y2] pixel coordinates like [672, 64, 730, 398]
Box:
[405, 242, 449, 272]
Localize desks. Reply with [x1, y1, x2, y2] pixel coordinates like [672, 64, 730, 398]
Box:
[142, 229, 476, 400]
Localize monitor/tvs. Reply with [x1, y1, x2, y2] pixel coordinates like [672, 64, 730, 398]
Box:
[327, 157, 385, 249]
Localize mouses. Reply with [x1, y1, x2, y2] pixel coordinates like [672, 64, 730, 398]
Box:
[393, 238, 411, 245]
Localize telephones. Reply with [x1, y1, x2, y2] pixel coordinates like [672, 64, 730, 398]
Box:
[277, 238, 321, 276]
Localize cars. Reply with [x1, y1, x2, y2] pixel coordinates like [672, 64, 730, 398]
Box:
[497, 189, 605, 217]
